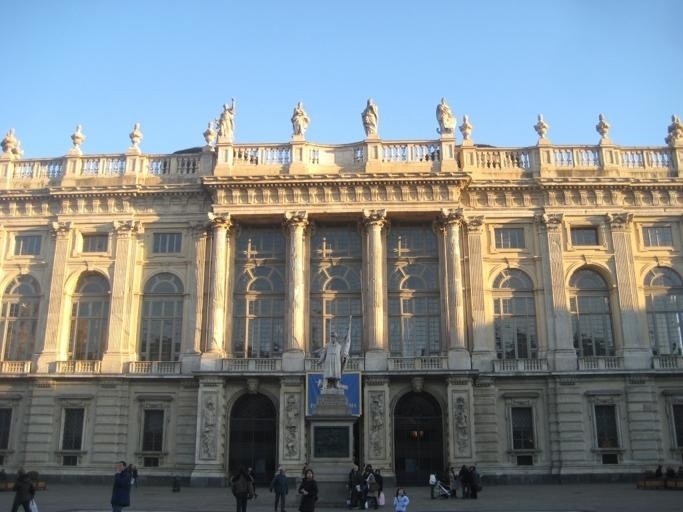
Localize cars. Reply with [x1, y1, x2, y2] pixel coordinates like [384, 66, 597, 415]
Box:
[420, 143, 524, 168]
[161, 145, 259, 174]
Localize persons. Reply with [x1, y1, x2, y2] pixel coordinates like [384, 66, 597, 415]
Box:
[204, 122, 217, 141]
[110, 459, 131, 512]
[127, 122, 144, 146]
[11, 468, 37, 511]
[429, 463, 481, 499]
[392, 487, 410, 512]
[667, 114, 683, 138]
[359, 96, 380, 130]
[318, 332, 349, 389]
[595, 113, 610, 133]
[229, 457, 384, 512]
[437, 96, 457, 134]
[127, 462, 132, 488]
[131, 467, 139, 488]
[458, 114, 473, 131]
[533, 113, 549, 134]
[71, 125, 85, 145]
[290, 100, 311, 134]
[216, 96, 237, 138]
[3, 127, 14, 154]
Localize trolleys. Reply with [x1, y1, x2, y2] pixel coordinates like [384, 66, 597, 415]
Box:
[436, 479, 458, 498]
